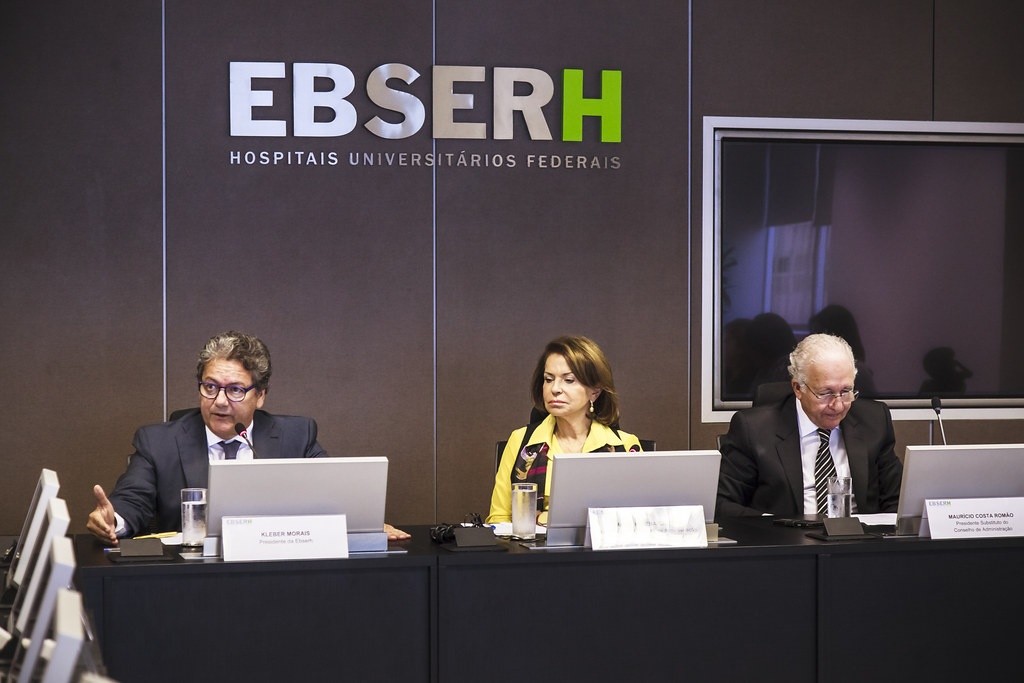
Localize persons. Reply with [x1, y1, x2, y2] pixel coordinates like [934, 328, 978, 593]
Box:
[483, 335, 643, 527]
[84, 330, 411, 548]
[711, 333, 904, 518]
[721, 305, 989, 399]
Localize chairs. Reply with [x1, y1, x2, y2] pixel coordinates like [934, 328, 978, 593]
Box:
[494, 439, 656, 475]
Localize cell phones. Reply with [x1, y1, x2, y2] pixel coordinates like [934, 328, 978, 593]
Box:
[773, 518, 823, 527]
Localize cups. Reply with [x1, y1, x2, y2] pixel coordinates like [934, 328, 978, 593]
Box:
[512, 483, 537, 539]
[827, 477, 852, 518]
[180, 487, 207, 546]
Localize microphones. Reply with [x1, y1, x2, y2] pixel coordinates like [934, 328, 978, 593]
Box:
[630, 444, 640, 452]
[930, 396, 946, 445]
[234, 423, 259, 459]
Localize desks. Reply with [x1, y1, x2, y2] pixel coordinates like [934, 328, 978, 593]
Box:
[67, 513, 1024, 683]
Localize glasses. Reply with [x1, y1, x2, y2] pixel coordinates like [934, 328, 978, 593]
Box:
[198, 376, 255, 403]
[800, 381, 860, 403]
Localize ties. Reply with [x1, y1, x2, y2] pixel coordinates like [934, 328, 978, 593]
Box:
[814, 427, 842, 515]
[217, 440, 242, 460]
